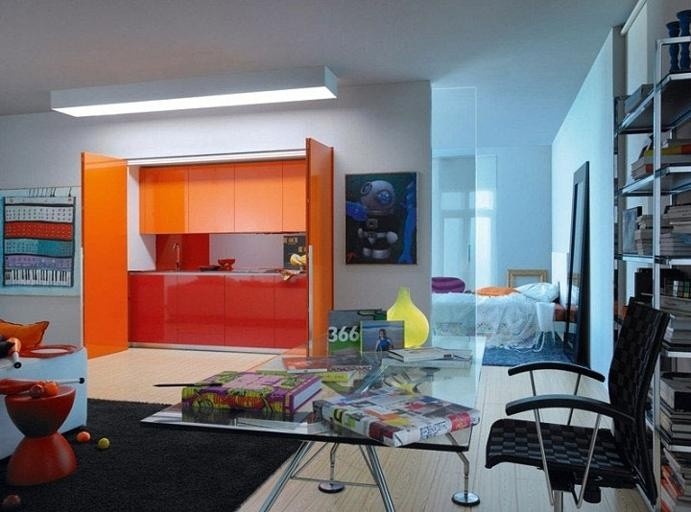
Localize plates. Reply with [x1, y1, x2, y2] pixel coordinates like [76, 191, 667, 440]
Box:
[26, 345, 78, 356]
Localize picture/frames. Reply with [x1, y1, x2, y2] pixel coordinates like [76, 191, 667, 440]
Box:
[505, 269, 546, 290]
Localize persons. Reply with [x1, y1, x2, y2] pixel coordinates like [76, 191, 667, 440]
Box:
[376, 328, 394, 350]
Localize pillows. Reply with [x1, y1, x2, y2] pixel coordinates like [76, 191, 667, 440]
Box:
[513, 282, 561, 302]
[477, 286, 516, 296]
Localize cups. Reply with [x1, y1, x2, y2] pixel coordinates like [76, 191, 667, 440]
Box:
[665, 9, 691, 74]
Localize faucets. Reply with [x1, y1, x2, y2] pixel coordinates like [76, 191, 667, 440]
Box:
[172, 241, 181, 272]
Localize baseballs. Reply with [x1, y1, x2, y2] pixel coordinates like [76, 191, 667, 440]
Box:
[3, 495, 20, 507]
[99, 438, 111, 449]
[78, 432, 90, 441]
[29, 383, 44, 399]
[47, 382, 60, 398]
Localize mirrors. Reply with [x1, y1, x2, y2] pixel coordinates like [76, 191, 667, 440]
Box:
[565, 162, 592, 358]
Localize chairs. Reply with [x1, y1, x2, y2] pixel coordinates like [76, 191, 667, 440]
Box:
[487, 295, 676, 510]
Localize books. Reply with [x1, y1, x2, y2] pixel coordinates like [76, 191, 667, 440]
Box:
[182, 370, 323, 418]
[313, 386, 480, 449]
[622, 82, 691, 511]
[382, 353, 474, 369]
[388, 346, 455, 363]
[256, 355, 354, 383]
[283, 349, 372, 372]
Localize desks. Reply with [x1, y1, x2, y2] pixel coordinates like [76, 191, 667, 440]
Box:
[142, 337, 486, 511]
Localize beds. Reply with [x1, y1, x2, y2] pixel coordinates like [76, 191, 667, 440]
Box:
[431, 283, 559, 352]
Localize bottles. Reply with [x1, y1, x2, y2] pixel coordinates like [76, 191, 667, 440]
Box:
[387, 286, 431, 349]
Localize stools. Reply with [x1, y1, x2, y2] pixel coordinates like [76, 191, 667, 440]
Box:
[0, 346, 85, 458]
[6, 383, 79, 485]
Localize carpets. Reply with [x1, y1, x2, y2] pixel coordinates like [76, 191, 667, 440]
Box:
[482, 332, 582, 365]
[0, 398, 313, 512]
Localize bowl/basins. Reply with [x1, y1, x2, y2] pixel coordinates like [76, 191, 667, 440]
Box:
[218, 259, 236, 272]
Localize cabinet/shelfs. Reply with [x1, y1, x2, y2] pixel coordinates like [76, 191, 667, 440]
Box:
[129, 274, 226, 349]
[137, 162, 235, 234]
[236, 159, 308, 233]
[227, 273, 312, 355]
[614, 0, 691, 512]
